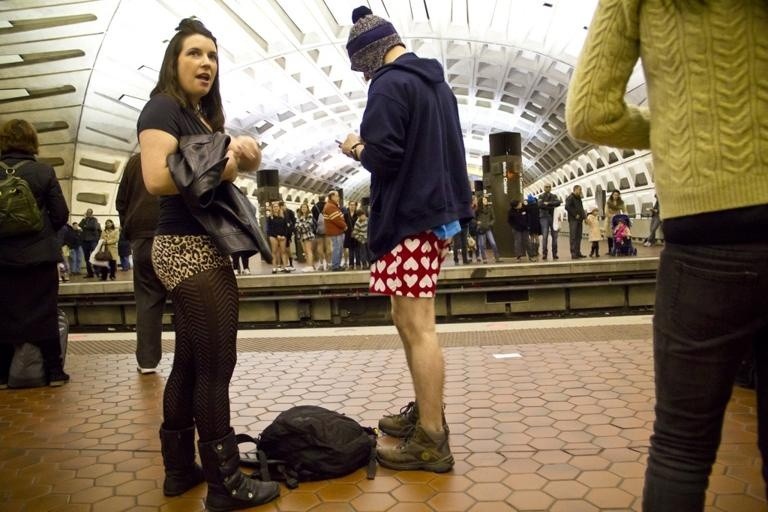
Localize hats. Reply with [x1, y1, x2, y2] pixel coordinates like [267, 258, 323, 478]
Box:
[345, 5, 406, 80]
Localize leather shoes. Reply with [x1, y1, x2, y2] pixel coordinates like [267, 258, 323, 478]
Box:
[49, 373, 69, 389]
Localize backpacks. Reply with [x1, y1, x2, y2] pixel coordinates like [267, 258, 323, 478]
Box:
[233, 405, 379, 490]
[7, 306, 69, 390]
[0, 159, 45, 234]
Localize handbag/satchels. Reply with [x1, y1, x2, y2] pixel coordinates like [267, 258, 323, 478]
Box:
[95, 241, 112, 260]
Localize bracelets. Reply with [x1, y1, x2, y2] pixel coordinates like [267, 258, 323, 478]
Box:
[350, 142, 365, 160]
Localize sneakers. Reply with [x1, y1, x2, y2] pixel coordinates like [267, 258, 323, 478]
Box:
[233, 269, 238, 276]
[243, 269, 250, 274]
[284, 267, 289, 273]
[272, 268, 276, 273]
[136, 365, 156, 376]
[377, 399, 449, 439]
[374, 424, 455, 474]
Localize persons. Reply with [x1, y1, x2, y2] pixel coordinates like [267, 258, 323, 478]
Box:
[453, 194, 503, 263]
[265, 190, 370, 273]
[136, 19, 282, 512]
[115, 153, 167, 376]
[61, 209, 131, 279]
[645, 193, 659, 246]
[334, 5, 474, 473]
[566, 185, 632, 258]
[1, 120, 69, 386]
[565, 0, 768, 511]
[507, 184, 561, 260]
[234, 256, 251, 274]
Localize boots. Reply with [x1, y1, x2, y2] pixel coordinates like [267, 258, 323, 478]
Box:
[197, 426, 280, 512]
[158, 420, 204, 498]
